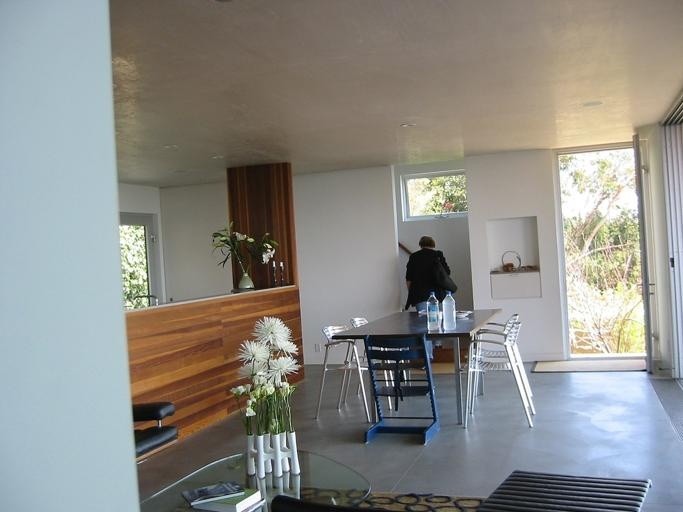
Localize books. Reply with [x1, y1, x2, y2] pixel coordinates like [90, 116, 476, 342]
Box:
[180, 479, 245, 507]
[241, 498, 265, 511]
[193, 481, 261, 512]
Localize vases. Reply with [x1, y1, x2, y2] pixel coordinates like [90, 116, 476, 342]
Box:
[245, 431, 301, 479]
[239, 272, 254, 289]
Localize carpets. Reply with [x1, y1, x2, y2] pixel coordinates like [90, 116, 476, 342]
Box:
[300, 487, 489, 512]
[531, 358, 646, 373]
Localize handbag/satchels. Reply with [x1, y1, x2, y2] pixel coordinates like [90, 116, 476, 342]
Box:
[435, 262, 457, 292]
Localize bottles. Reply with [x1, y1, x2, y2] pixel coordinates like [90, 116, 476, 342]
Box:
[426, 290, 440, 330]
[440, 290, 457, 330]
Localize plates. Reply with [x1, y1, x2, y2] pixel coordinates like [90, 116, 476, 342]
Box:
[455, 311, 474, 319]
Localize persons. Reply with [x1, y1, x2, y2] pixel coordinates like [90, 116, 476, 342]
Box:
[404, 236, 457, 349]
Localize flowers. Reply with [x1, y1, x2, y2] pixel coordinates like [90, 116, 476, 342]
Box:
[230, 316, 300, 435]
[212, 221, 278, 271]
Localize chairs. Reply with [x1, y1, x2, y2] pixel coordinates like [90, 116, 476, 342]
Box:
[316, 314, 536, 445]
[133, 402, 179, 464]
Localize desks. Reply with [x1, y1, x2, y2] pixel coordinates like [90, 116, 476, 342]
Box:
[332, 308, 501, 425]
[139, 448, 371, 512]
